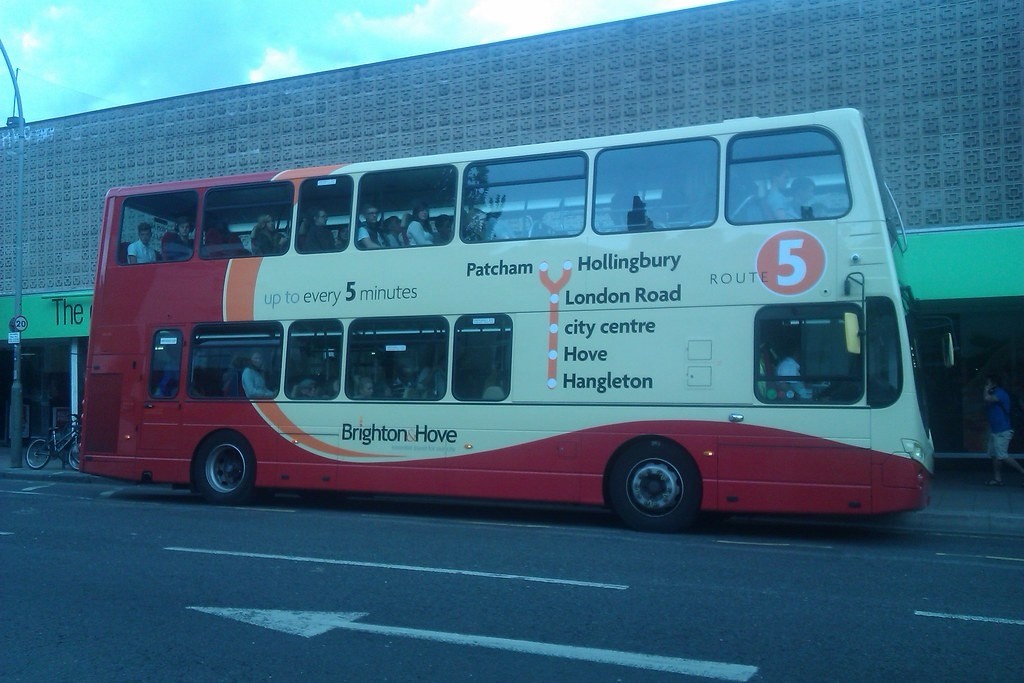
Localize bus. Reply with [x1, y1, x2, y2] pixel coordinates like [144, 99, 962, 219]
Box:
[81, 109, 935, 534]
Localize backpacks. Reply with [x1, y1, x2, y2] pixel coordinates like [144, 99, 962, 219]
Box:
[997, 401, 1023, 427]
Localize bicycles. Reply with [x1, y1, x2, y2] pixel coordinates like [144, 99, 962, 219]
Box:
[26, 413, 81, 470]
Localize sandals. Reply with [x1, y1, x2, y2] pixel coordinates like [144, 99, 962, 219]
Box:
[984, 479, 1005, 486]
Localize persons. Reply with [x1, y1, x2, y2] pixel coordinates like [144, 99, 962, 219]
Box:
[240, 348, 279, 398]
[165, 216, 195, 261]
[609, 177, 645, 230]
[358, 202, 452, 249]
[370, 351, 446, 400]
[352, 374, 377, 401]
[483, 201, 516, 241]
[298, 380, 330, 400]
[774, 349, 831, 400]
[319, 355, 340, 394]
[127, 222, 156, 265]
[303, 207, 350, 253]
[980, 373, 1024, 487]
[764, 164, 816, 222]
[251, 214, 290, 257]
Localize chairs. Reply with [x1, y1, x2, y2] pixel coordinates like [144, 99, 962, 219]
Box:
[114, 212, 555, 266]
[764, 345, 783, 396]
[403, 389, 415, 400]
[291, 385, 300, 400]
[482, 387, 503, 400]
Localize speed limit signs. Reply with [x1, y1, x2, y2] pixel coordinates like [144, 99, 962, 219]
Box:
[15, 316, 28, 331]
